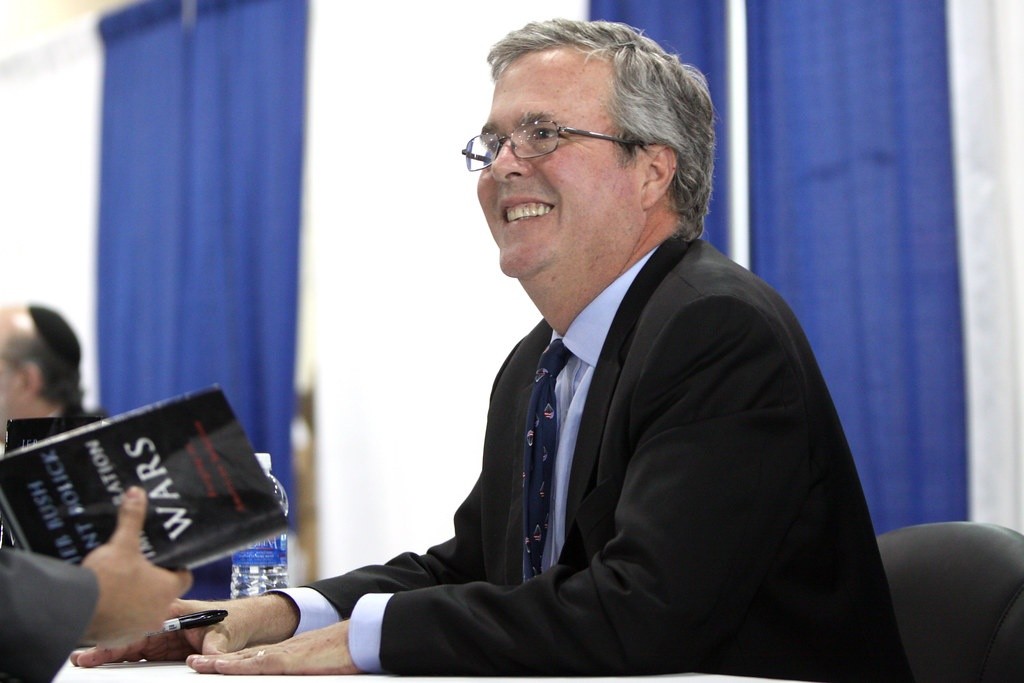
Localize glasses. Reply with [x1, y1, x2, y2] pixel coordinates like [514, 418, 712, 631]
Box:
[461, 120, 644, 172]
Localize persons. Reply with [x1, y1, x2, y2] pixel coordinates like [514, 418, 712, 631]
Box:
[0, 305, 194, 683]
[71, 17, 915, 683]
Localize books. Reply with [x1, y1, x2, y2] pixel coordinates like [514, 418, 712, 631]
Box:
[0, 381, 291, 575]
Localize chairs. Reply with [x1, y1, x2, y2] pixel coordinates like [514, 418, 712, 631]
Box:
[875, 522, 1021, 681]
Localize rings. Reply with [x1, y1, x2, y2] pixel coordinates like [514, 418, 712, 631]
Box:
[256, 648, 267, 658]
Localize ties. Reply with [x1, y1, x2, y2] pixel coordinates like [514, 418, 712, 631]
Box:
[522, 339, 572, 582]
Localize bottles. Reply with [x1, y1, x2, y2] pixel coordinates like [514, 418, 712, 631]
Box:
[230, 452, 290, 600]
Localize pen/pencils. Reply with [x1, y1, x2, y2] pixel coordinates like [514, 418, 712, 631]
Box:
[144, 609, 228, 636]
